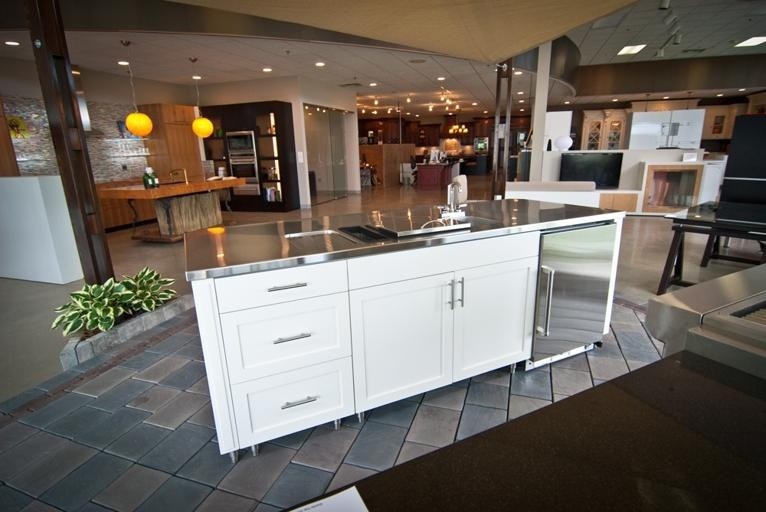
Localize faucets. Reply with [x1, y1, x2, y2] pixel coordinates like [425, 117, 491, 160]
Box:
[168, 168, 189, 185]
[440, 180, 466, 220]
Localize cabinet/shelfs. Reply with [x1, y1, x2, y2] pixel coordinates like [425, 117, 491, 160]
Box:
[523, 198, 628, 374]
[356, 203, 542, 425]
[182, 214, 356, 463]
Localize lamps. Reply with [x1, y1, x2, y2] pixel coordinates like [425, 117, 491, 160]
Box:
[118, 107, 159, 137]
[188, 113, 216, 140]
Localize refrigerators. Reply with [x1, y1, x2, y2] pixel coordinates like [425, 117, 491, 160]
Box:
[629, 108, 705, 150]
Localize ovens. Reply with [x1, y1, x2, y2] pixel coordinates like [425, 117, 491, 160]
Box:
[225, 131, 260, 196]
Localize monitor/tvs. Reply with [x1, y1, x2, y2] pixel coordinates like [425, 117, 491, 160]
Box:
[560, 152, 623, 189]
